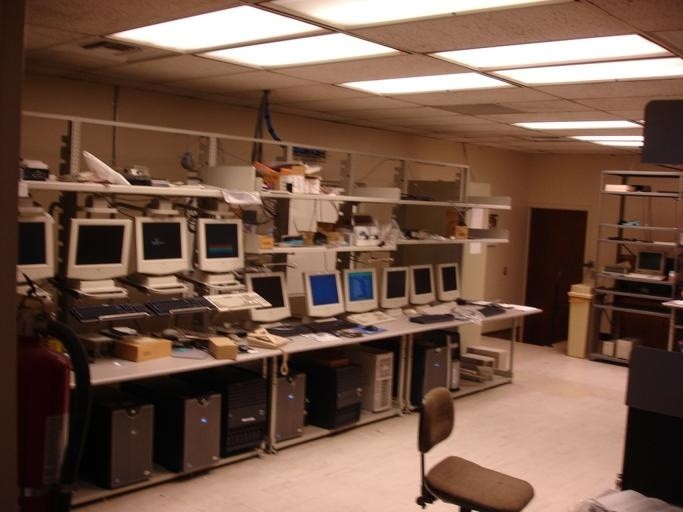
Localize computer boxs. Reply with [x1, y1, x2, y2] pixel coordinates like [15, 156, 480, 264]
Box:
[86, 330, 460, 489]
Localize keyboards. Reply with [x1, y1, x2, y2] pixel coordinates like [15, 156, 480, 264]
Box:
[346, 311, 397, 326]
[410, 313, 454, 323]
[265, 324, 313, 337]
[624, 272, 668, 281]
[70, 303, 154, 322]
[145, 296, 218, 317]
[477, 307, 505, 317]
[202, 291, 272, 313]
[303, 319, 359, 334]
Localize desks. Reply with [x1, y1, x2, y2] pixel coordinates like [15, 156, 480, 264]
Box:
[74, 301, 543, 504]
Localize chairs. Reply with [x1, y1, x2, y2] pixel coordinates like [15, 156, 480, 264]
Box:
[416, 387, 535, 512]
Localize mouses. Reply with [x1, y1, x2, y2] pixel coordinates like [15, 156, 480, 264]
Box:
[363, 325, 377, 330]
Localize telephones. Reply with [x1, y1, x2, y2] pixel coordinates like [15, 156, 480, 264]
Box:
[246, 328, 288, 349]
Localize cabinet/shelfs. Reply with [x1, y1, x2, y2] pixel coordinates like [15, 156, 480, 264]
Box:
[591, 170, 683, 368]
[17, 110, 512, 297]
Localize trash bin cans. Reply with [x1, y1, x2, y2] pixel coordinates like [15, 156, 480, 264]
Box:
[567, 284, 607, 358]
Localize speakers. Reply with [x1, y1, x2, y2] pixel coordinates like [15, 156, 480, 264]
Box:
[640, 99, 683, 164]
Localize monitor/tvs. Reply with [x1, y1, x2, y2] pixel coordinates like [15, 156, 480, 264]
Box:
[379, 266, 409, 316]
[64, 218, 133, 299]
[635, 249, 667, 275]
[245, 271, 292, 329]
[134, 216, 188, 295]
[343, 268, 379, 313]
[303, 272, 345, 323]
[197, 217, 245, 290]
[409, 264, 437, 311]
[16, 212, 55, 297]
[435, 262, 460, 305]
